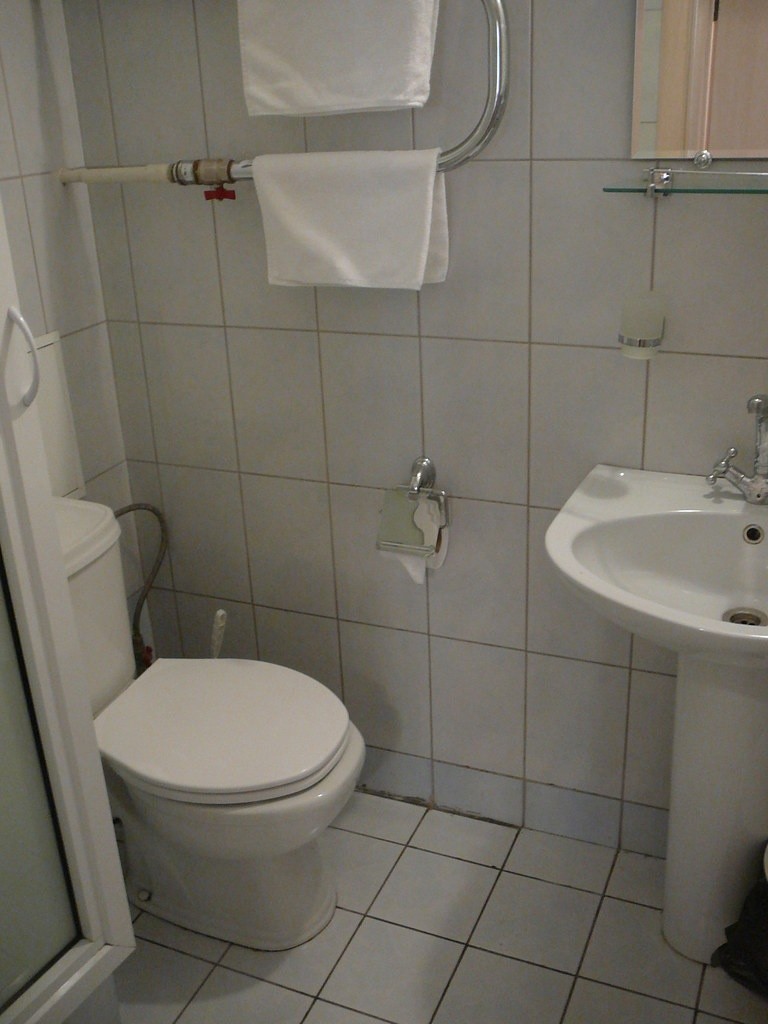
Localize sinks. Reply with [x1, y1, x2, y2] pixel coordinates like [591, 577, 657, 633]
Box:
[541, 461, 768, 670]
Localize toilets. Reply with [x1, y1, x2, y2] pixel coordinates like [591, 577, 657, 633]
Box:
[54, 490, 371, 954]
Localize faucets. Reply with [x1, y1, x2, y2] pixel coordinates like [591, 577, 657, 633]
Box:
[707, 391, 768, 508]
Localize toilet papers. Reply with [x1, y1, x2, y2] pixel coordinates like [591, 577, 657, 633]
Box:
[378, 500, 451, 585]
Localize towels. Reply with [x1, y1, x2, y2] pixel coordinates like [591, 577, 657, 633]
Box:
[249, 144, 449, 293]
[235, 0, 441, 119]
